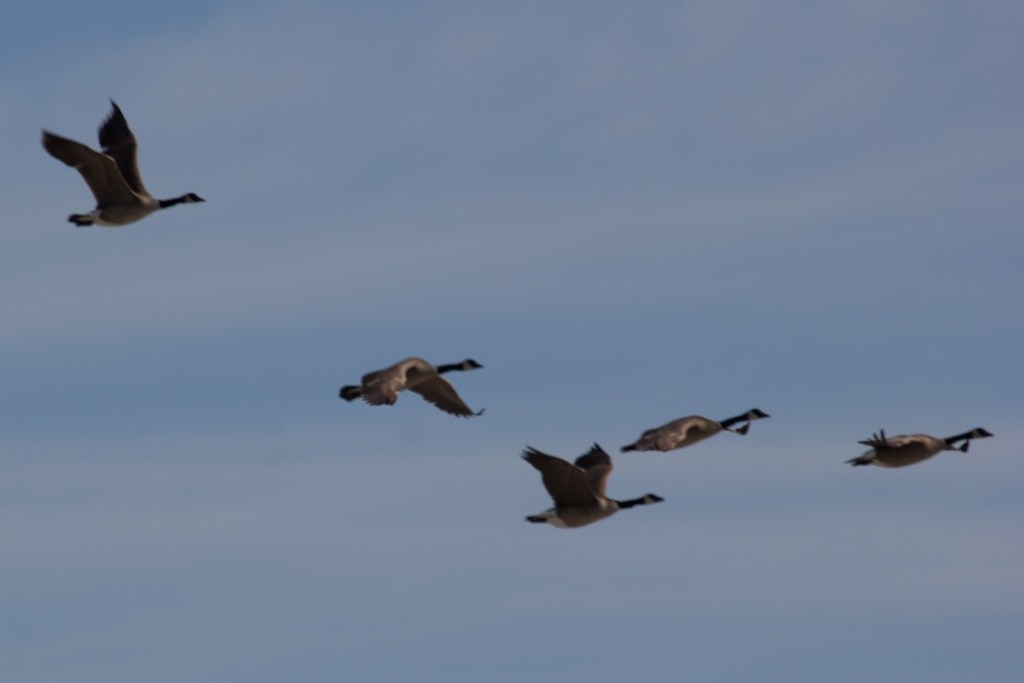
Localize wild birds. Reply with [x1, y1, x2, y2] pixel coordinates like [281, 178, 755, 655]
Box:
[339, 357, 487, 420]
[619, 408, 772, 455]
[42, 96, 207, 227]
[844, 427, 994, 468]
[520, 440, 666, 530]
